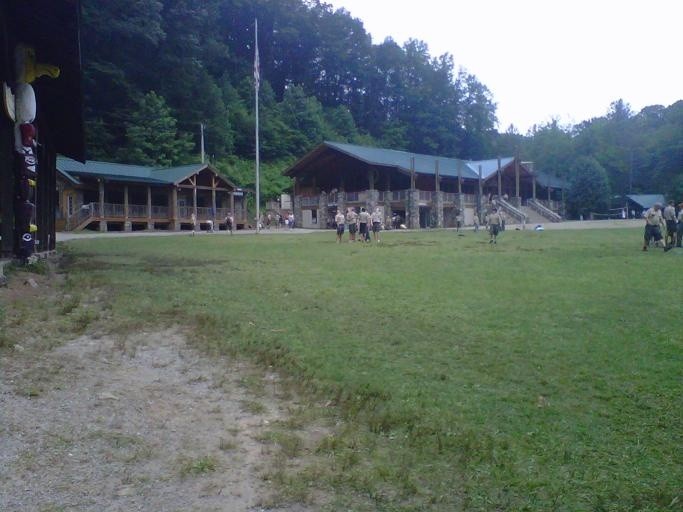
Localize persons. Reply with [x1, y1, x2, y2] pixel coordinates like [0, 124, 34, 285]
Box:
[356, 207, 368, 242]
[369, 208, 383, 241]
[258, 211, 296, 231]
[485, 207, 501, 244]
[345, 207, 358, 242]
[333, 210, 343, 246]
[662, 200, 676, 245]
[472, 212, 479, 231]
[641, 202, 670, 253]
[455, 212, 462, 228]
[223, 212, 234, 234]
[675, 202, 683, 247]
